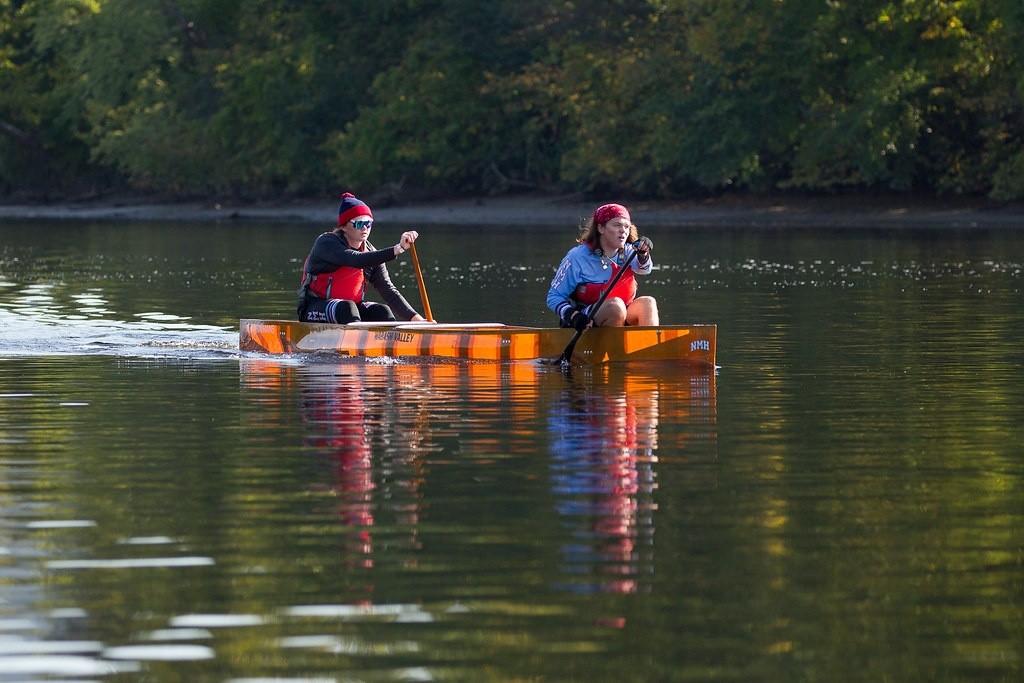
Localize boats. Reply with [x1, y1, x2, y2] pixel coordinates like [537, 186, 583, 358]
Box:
[237, 319, 717, 372]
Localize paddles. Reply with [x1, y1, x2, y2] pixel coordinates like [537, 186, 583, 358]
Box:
[545, 242, 642, 367]
[403, 234, 438, 327]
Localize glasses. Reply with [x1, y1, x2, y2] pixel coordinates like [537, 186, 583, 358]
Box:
[348, 220, 373, 229]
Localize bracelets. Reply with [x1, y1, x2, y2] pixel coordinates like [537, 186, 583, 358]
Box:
[396, 243, 405, 253]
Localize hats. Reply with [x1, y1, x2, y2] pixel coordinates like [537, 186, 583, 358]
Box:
[338, 193, 373, 228]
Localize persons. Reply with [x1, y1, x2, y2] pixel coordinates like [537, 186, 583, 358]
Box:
[546, 204, 659, 333]
[297, 193, 437, 324]
